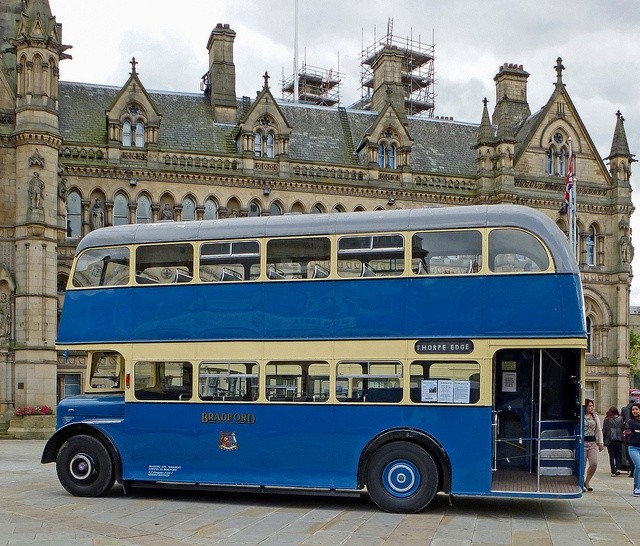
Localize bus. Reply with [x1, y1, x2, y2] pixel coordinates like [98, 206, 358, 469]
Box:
[40, 203, 588, 513]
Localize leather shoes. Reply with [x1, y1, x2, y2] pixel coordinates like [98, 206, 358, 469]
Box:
[584, 483, 594, 491]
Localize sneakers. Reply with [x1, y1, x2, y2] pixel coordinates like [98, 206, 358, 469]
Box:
[633, 489, 640, 497]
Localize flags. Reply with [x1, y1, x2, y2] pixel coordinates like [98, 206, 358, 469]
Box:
[558, 152, 576, 216]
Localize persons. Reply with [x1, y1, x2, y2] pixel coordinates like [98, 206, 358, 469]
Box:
[620, 400, 640, 498]
[604, 406, 624, 478]
[582, 399, 604, 492]
